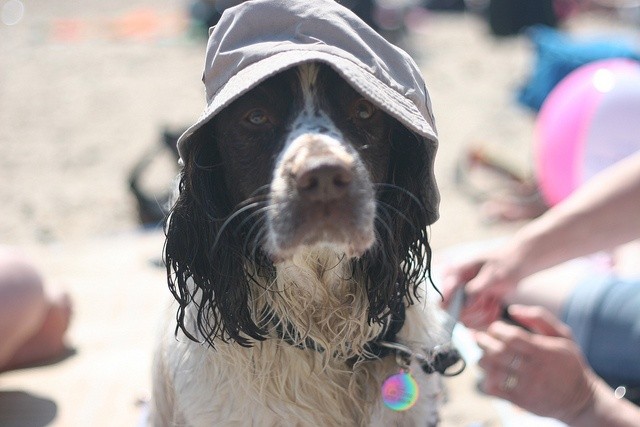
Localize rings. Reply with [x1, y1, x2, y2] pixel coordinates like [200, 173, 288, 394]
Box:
[501, 373, 521, 394]
[510, 353, 525, 369]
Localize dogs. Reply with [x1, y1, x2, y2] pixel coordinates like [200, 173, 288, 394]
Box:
[144, 1, 455, 426]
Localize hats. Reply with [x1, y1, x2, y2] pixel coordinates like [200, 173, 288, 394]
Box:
[177, 0, 441, 226]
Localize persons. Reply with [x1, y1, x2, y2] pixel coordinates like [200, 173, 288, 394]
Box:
[0, 256, 73, 369]
[438, 150, 640, 426]
